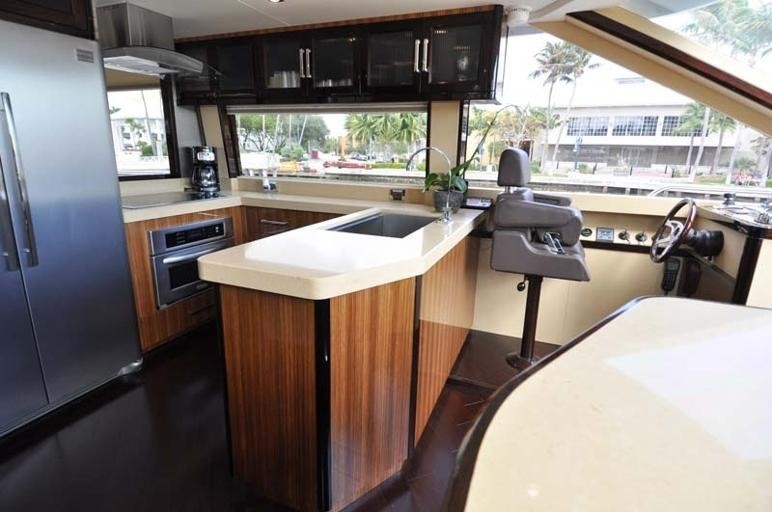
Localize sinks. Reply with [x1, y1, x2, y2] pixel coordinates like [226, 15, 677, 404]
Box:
[326, 207, 444, 239]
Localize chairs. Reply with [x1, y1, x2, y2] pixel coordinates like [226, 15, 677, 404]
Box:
[489, 148, 591, 371]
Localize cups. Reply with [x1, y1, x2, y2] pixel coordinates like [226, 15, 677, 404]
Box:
[267, 69, 353, 88]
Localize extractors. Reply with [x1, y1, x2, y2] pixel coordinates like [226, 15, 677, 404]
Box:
[95, 0, 204, 80]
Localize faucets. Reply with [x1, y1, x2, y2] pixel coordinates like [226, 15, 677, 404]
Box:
[403, 145, 454, 225]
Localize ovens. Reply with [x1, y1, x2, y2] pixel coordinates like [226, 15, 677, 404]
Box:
[147, 215, 236, 312]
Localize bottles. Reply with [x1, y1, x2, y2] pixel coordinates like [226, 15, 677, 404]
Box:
[262, 170, 271, 192]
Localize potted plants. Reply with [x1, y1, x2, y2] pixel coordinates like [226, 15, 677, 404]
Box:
[422, 157, 480, 214]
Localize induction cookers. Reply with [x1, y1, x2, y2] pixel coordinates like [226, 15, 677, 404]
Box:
[120, 190, 228, 209]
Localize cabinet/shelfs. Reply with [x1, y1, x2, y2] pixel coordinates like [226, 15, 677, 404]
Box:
[173, 29, 259, 106]
[252, 18, 361, 105]
[362, 5, 509, 105]
[243, 205, 346, 244]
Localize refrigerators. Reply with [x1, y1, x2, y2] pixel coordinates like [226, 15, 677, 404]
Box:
[0, 17, 146, 443]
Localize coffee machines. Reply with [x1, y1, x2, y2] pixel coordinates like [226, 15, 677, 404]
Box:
[190, 143, 220, 193]
